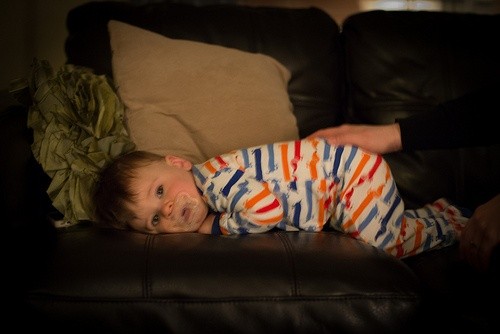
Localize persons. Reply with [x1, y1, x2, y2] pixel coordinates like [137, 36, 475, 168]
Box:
[91, 138, 472, 260]
[303, 93, 500, 270]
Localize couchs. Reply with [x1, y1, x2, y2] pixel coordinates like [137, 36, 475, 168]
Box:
[0, 0, 500, 334]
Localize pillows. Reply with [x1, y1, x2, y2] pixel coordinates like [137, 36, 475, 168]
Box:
[108, 19, 298, 165]
